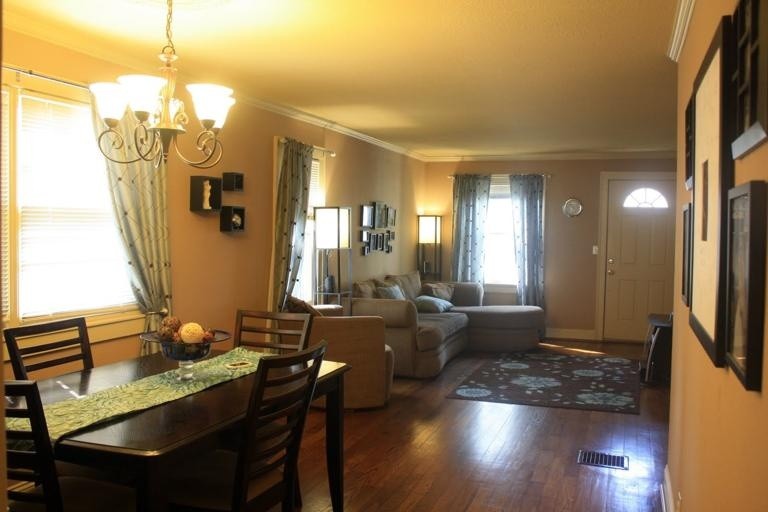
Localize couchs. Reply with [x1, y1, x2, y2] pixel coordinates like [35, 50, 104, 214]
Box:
[330, 280, 545, 381]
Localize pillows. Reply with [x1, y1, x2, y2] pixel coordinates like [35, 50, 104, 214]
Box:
[286, 294, 324, 318]
[350, 270, 454, 314]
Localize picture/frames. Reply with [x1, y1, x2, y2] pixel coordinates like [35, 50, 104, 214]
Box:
[678, 0, 767, 395]
[359, 200, 397, 257]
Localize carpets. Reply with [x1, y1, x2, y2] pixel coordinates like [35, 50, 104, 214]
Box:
[445, 338, 642, 415]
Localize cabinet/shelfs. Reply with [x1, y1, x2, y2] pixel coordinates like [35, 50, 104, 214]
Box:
[188, 171, 245, 232]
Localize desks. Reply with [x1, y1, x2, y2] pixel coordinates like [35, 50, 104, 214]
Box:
[1, 348, 354, 512]
[641, 313, 671, 389]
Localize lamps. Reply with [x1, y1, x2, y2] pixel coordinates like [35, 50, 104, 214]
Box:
[313, 206, 352, 290]
[86, 0, 236, 169]
[416, 214, 443, 275]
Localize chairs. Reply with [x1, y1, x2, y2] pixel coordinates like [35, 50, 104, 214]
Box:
[207, 307, 313, 509]
[2, 379, 138, 512]
[2, 316, 130, 491]
[275, 304, 394, 413]
[150, 337, 328, 512]
